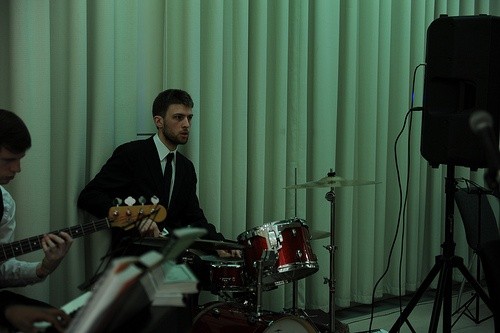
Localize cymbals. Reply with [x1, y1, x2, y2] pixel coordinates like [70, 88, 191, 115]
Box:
[309, 228, 330, 241]
[279, 177, 382, 189]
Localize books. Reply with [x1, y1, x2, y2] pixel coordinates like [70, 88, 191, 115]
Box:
[64, 226, 207, 333]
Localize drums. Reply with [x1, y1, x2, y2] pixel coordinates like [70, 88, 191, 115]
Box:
[190, 301, 318, 333]
[237, 218, 319, 292]
[198, 255, 250, 292]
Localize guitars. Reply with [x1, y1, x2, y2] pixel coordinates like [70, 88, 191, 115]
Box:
[0, 195, 167, 266]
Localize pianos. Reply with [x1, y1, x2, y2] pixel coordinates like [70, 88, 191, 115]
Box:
[35, 289, 93, 333]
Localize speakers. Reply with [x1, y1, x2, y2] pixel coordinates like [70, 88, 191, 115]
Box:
[421, 14, 500, 173]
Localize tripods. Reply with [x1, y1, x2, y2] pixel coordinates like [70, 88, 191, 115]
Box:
[387, 167, 500, 333]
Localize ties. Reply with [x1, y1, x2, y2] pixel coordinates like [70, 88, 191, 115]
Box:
[164, 152, 175, 210]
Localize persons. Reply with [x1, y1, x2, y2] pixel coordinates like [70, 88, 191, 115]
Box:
[77, 89, 241, 259]
[0, 109, 74, 289]
